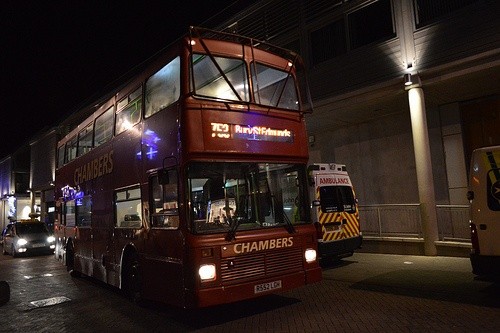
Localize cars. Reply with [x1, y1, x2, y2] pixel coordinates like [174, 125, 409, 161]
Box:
[1, 220, 55, 258]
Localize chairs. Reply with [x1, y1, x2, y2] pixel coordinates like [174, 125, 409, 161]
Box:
[156, 207, 180, 229]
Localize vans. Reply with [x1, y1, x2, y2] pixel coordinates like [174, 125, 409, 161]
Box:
[465, 146, 500, 280]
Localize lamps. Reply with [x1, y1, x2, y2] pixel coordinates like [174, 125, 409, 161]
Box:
[404, 73, 413, 86]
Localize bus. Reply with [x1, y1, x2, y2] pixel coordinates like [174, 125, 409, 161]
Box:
[136, 161, 364, 270]
[50, 24, 325, 313]
